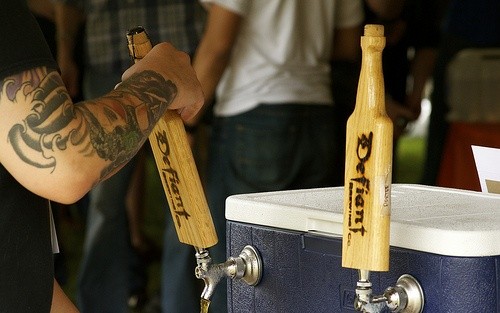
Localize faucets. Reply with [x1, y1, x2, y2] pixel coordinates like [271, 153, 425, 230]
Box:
[127, 28, 263, 303]
[341, 21, 425, 313]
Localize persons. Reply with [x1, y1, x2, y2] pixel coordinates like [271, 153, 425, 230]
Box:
[0, 0, 204, 313]
[54, 0, 365, 313]
[332, 0, 500, 183]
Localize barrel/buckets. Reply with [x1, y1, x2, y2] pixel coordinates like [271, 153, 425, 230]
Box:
[437, 47, 500, 191]
